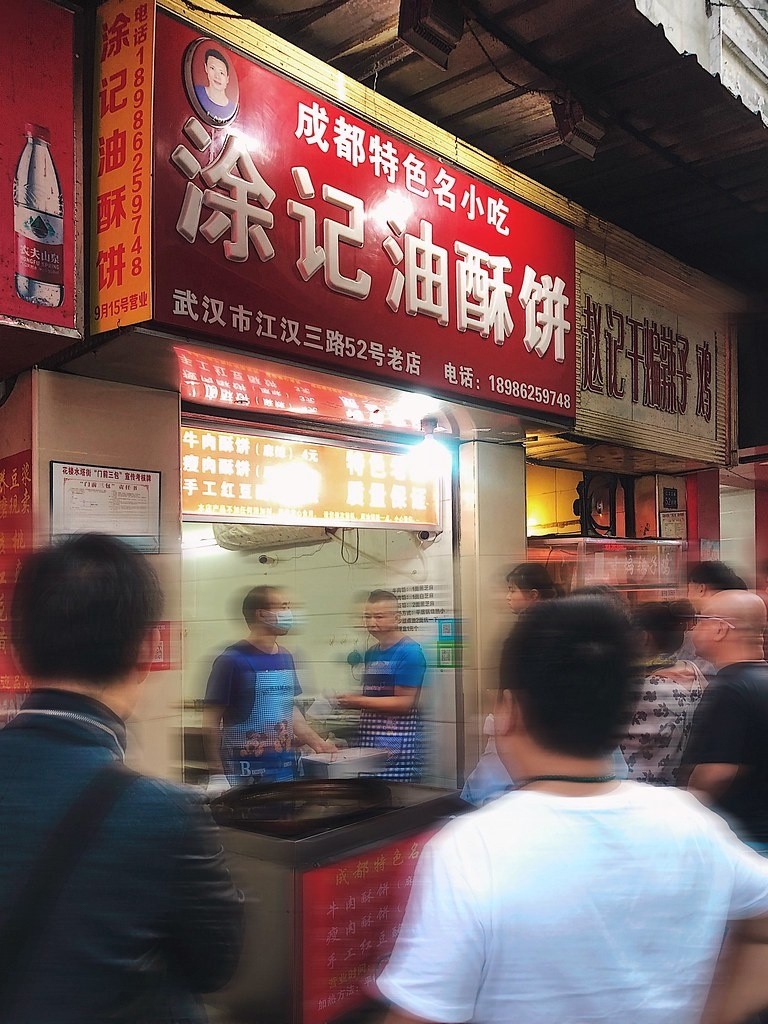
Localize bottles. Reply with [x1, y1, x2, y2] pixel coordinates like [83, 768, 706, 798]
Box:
[12, 123, 65, 308]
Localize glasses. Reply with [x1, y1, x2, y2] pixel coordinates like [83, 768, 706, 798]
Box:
[693, 614, 735, 629]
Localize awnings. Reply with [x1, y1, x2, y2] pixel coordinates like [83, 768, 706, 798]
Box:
[233, 0, 766, 306]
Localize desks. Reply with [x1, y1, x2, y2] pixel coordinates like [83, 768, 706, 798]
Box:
[182, 716, 358, 772]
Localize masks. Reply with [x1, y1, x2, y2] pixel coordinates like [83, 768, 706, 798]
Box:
[256, 609, 294, 631]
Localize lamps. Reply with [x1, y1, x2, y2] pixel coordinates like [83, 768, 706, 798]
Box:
[397, 0, 465, 72]
[551, 98, 607, 163]
[411, 417, 453, 480]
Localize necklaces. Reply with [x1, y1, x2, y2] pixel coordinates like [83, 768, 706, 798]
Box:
[503, 769, 617, 794]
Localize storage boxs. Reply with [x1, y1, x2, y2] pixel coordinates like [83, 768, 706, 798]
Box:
[303, 744, 390, 778]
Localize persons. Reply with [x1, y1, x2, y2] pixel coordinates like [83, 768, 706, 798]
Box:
[676, 589, 766, 859]
[665, 560, 739, 682]
[506, 563, 566, 613]
[194, 48, 236, 121]
[1, 533, 245, 1024]
[337, 590, 426, 784]
[373, 594, 767, 1024]
[620, 599, 708, 787]
[204, 586, 337, 797]
